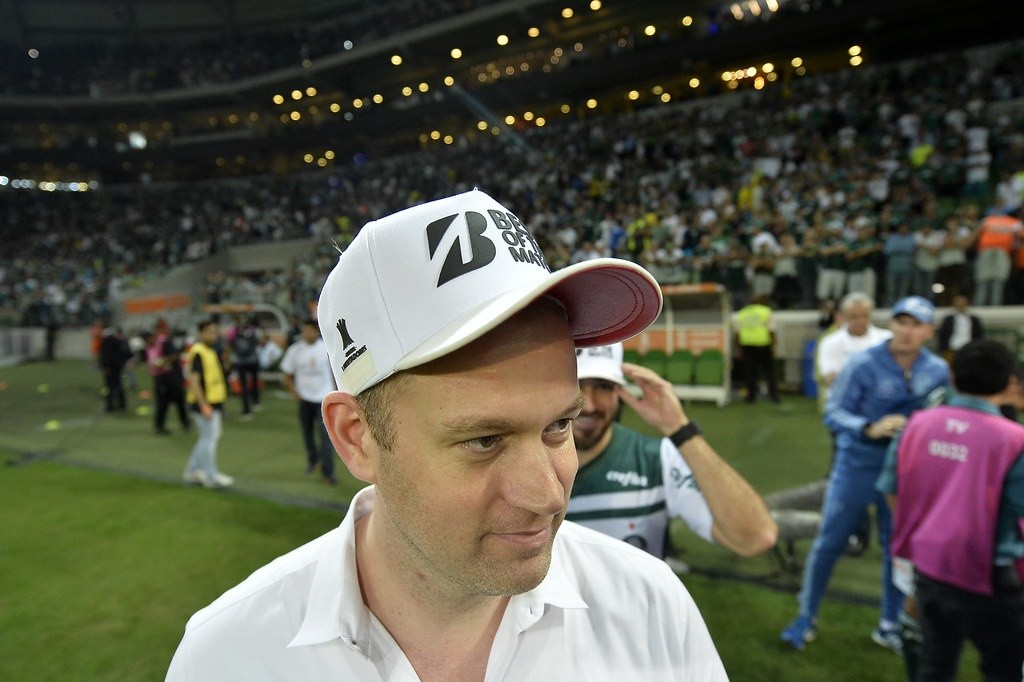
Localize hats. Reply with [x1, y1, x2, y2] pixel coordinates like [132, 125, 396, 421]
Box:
[317, 190, 663, 397]
[892, 295, 936, 326]
[574, 338, 626, 388]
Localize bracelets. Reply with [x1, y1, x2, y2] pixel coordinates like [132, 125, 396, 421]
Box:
[669, 419, 701, 447]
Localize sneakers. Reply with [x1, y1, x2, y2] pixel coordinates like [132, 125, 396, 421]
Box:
[780, 617, 819, 650]
[871, 624, 905, 657]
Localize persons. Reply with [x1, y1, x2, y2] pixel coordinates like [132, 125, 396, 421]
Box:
[0, 39, 1024, 682]
[163, 189, 732, 682]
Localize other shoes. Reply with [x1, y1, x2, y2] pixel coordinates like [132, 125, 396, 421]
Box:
[307, 460, 315, 472]
[324, 473, 337, 485]
[182, 470, 204, 483]
[203, 474, 233, 489]
